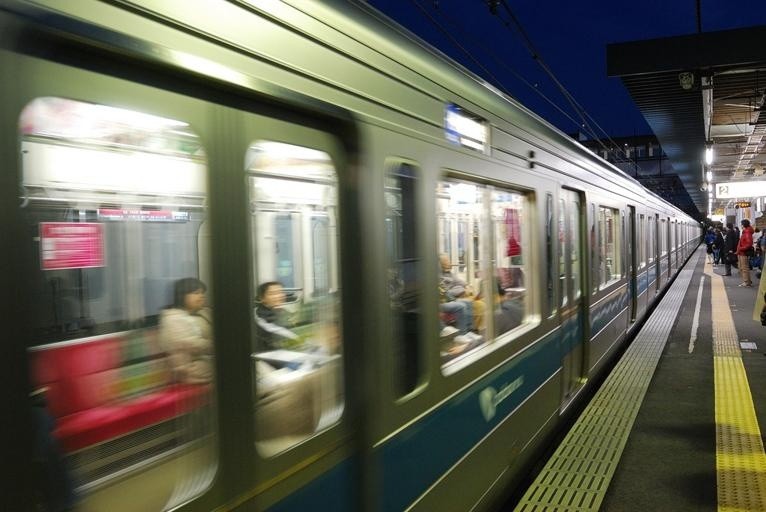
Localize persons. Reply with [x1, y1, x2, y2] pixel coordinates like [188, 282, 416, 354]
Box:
[386, 247, 615, 356]
[701, 219, 766, 325]
[156, 276, 302, 395]
[254, 282, 327, 357]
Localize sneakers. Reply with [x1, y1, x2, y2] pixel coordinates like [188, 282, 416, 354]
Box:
[440, 325, 483, 344]
[738, 282, 751, 287]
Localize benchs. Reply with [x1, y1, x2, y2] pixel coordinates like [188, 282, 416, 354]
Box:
[26, 327, 213, 469]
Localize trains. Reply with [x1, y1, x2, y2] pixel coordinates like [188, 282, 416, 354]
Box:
[0, 2, 707, 511]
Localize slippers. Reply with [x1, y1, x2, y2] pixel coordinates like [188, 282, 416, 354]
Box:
[721, 274, 731, 276]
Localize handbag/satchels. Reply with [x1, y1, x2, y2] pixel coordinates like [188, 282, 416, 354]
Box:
[744, 247, 754, 257]
[725, 253, 738, 265]
[174, 354, 215, 379]
